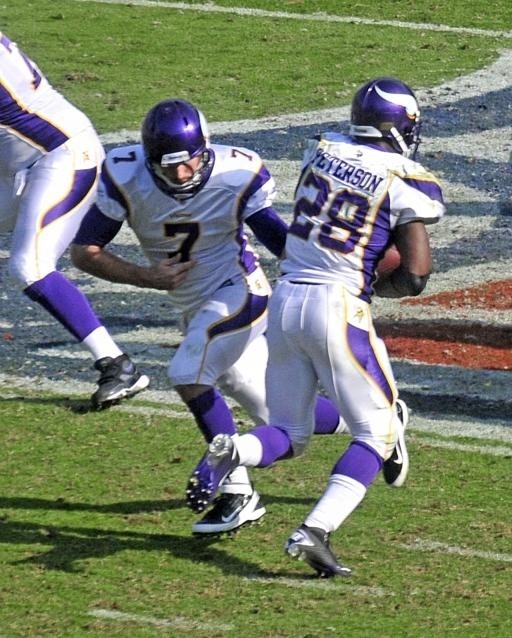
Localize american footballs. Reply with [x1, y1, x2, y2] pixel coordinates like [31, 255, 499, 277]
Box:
[375, 245, 401, 278]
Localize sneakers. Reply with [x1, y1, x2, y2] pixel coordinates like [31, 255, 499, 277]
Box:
[192, 482, 267, 536]
[382, 399, 410, 487]
[285, 523, 352, 577]
[91, 353, 151, 406]
[186, 433, 240, 514]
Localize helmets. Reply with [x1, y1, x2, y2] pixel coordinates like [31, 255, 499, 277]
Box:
[141, 100, 212, 200]
[350, 79, 422, 161]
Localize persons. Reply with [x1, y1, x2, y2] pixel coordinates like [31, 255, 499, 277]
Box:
[72, 97, 411, 539]
[0, 31, 150, 407]
[182, 76, 445, 580]
